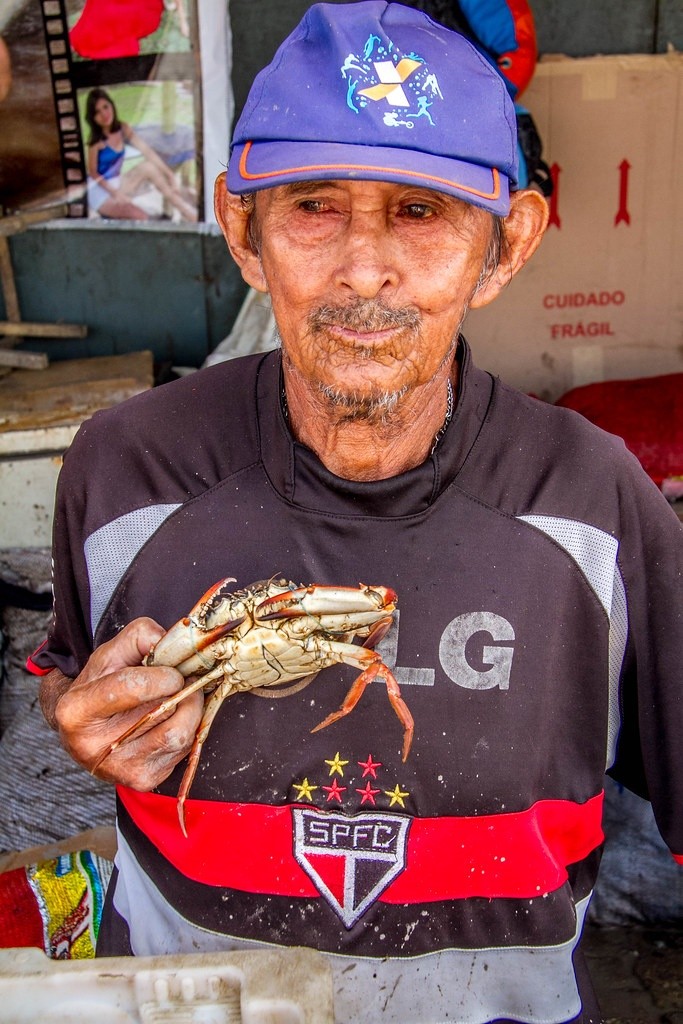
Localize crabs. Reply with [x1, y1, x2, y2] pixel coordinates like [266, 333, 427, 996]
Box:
[90, 571, 414, 839]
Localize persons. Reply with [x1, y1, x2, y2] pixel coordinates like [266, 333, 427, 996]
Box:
[32, 1, 683, 1020]
[81, 85, 201, 223]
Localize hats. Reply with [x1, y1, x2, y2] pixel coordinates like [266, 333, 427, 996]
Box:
[225, 0, 520, 218]
